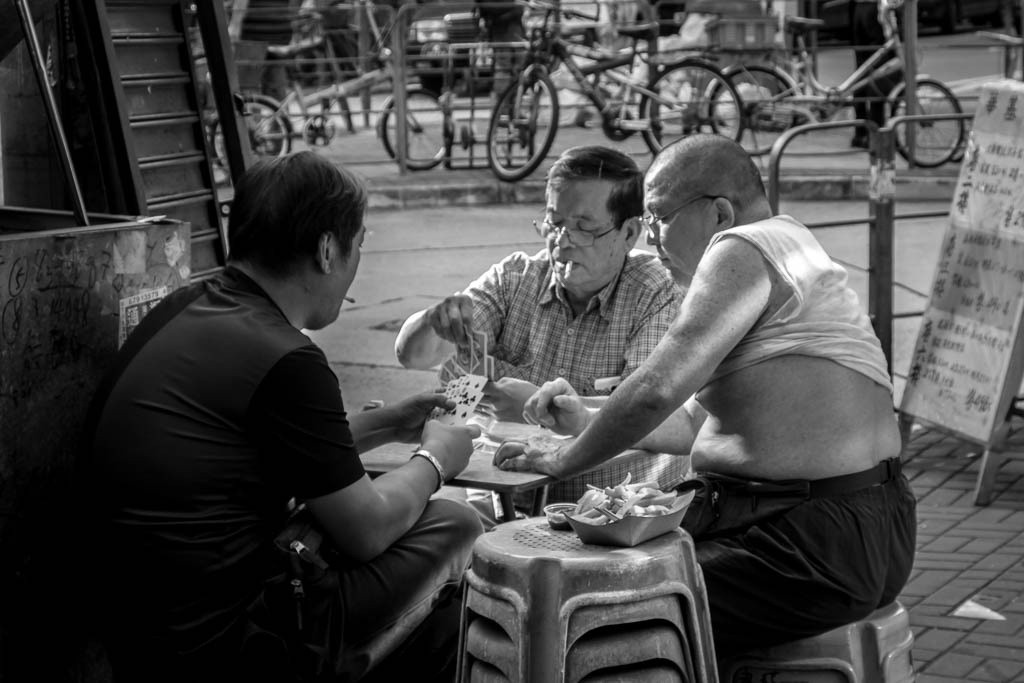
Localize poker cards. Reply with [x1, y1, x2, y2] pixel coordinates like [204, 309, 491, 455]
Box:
[425, 323, 507, 453]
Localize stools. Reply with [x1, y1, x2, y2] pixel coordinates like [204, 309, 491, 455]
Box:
[725, 593, 917, 683]
[453, 516, 720, 683]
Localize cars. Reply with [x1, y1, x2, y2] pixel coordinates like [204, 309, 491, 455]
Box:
[402, 0, 613, 95]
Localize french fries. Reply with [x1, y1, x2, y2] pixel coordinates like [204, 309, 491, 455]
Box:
[586, 472, 677, 522]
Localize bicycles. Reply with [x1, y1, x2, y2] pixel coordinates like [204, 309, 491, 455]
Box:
[204, 0, 455, 178]
[487, 0, 746, 182]
[698, 0, 966, 169]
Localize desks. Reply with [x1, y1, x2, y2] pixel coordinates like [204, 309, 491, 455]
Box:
[357, 418, 653, 520]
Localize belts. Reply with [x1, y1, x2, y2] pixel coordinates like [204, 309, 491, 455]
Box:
[698, 457, 902, 501]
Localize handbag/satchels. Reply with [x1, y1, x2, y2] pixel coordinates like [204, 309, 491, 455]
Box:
[668, 477, 717, 535]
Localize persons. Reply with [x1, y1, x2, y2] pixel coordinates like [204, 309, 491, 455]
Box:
[395, 147, 689, 531]
[478, 0, 531, 129]
[87, 150, 486, 683]
[850, 0, 906, 150]
[228, 0, 297, 134]
[495, 134, 915, 658]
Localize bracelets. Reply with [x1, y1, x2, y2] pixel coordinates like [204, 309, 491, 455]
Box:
[408, 450, 446, 492]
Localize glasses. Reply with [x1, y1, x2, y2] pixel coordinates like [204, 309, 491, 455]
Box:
[638, 193, 727, 239]
[533, 221, 618, 248]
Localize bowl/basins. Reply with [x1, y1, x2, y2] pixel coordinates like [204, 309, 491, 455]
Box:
[543, 502, 578, 531]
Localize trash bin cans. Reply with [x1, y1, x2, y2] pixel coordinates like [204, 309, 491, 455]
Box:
[705, 15, 782, 99]
